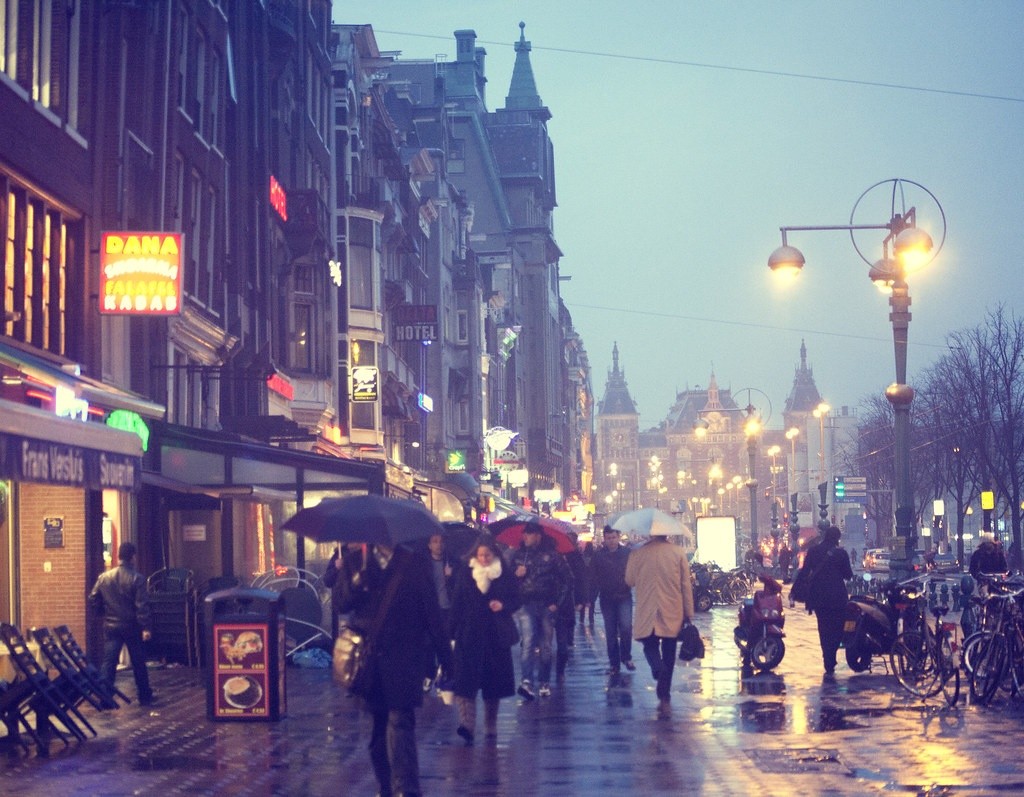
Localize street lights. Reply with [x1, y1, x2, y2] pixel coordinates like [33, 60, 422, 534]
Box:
[726, 482, 734, 517]
[785, 427, 800, 583]
[717, 488, 726, 517]
[732, 475, 742, 533]
[606, 463, 636, 512]
[766, 177, 948, 679]
[812, 402, 830, 541]
[678, 447, 724, 518]
[693, 387, 773, 576]
[767, 444, 783, 580]
[648, 456, 662, 510]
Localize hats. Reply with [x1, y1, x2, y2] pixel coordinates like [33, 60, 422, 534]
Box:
[522, 524, 542, 536]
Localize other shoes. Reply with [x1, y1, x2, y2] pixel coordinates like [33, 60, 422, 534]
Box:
[458, 726, 474, 743]
[556, 669, 566, 685]
[540, 684, 552, 697]
[652, 660, 661, 681]
[624, 659, 635, 671]
[611, 665, 620, 675]
[485, 732, 497, 742]
[138, 694, 158, 704]
[101, 700, 119, 709]
[518, 684, 535, 700]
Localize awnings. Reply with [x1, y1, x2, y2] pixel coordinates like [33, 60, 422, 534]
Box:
[0, 397, 140, 491]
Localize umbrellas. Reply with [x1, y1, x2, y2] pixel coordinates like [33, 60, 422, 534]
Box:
[280, 494, 440, 575]
[441, 519, 493, 568]
[605, 506, 693, 537]
[489, 513, 581, 567]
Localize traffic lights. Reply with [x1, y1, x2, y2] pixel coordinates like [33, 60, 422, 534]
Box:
[833, 476, 844, 503]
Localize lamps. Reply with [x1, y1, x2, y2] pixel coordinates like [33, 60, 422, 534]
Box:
[483, 425, 519, 452]
[158, 339, 280, 381]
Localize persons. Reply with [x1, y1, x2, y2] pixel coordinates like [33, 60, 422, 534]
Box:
[424, 534, 453, 698]
[568, 533, 598, 645]
[510, 523, 571, 700]
[789, 526, 852, 682]
[851, 548, 857, 562]
[625, 536, 695, 719]
[588, 526, 635, 675]
[745, 547, 763, 568]
[969, 533, 1008, 596]
[779, 542, 790, 584]
[555, 551, 586, 685]
[324, 543, 454, 797]
[444, 536, 520, 743]
[87, 542, 158, 705]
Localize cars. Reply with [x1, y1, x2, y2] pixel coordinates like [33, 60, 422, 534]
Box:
[911, 548, 927, 573]
[931, 553, 961, 574]
[863, 548, 891, 569]
[868, 551, 891, 573]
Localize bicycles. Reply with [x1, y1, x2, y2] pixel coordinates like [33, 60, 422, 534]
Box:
[888, 567, 1024, 716]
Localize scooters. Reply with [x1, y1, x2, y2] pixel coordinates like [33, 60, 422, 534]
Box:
[840, 549, 945, 674]
[689, 536, 801, 614]
[733, 569, 786, 671]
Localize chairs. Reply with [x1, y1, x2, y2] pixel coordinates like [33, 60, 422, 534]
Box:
[1, 622, 132, 756]
[145, 566, 244, 667]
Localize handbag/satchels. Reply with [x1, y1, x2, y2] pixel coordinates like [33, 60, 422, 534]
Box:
[495, 614, 520, 649]
[676, 620, 703, 661]
[794, 577, 811, 603]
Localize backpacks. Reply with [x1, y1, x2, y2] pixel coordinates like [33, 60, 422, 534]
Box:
[332, 627, 366, 691]
